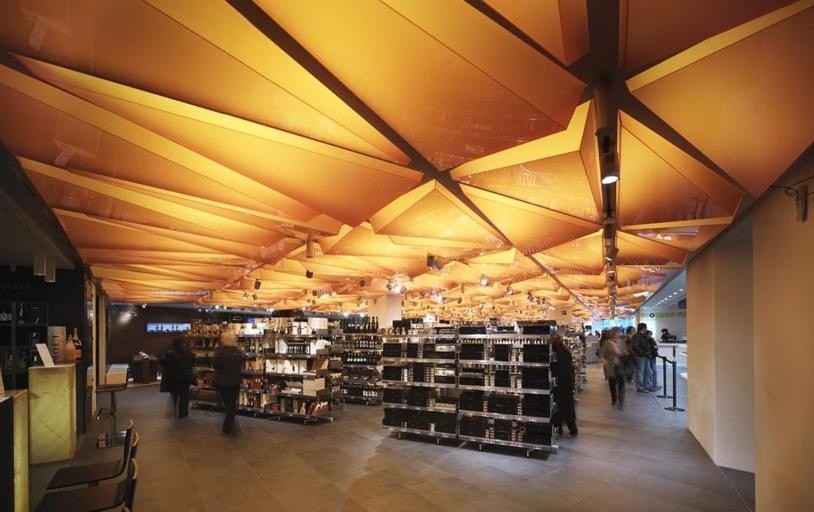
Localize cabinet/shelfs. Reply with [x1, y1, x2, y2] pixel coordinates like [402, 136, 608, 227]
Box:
[186, 330, 561, 456]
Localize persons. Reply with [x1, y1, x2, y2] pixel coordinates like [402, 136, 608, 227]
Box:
[215, 332, 243, 432]
[551, 336, 577, 435]
[596, 323, 663, 412]
[660, 328, 671, 343]
[162, 338, 196, 418]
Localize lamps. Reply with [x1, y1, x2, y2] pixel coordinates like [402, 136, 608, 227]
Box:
[603, 246, 619, 262]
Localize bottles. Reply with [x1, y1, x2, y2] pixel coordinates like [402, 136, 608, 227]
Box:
[238, 383, 253, 407]
[1, 302, 25, 325]
[334, 317, 383, 397]
[66, 327, 82, 360]
[286, 340, 309, 354]
[34, 355, 37, 364]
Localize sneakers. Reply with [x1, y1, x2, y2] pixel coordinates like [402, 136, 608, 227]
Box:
[637, 386, 662, 393]
[613, 398, 617, 405]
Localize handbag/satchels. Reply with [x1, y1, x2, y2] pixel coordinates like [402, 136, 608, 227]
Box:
[160, 376, 168, 392]
[213, 373, 240, 389]
[619, 356, 636, 376]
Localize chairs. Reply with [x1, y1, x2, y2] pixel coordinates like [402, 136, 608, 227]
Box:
[34, 367, 140, 511]
